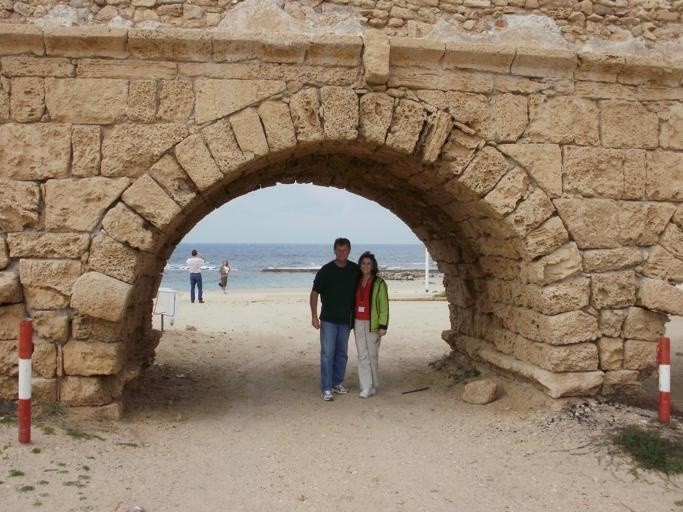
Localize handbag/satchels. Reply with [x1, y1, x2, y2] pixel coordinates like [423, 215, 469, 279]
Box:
[219, 282, 223, 287]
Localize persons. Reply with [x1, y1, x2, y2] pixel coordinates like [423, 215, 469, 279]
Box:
[185, 249, 204, 303]
[218, 259, 229, 292]
[308, 236, 359, 402]
[351, 249, 388, 397]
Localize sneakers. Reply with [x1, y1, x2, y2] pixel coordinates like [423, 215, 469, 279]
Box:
[320, 390, 335, 401]
[332, 384, 349, 393]
[359, 388, 376, 398]
[191, 300, 204, 303]
